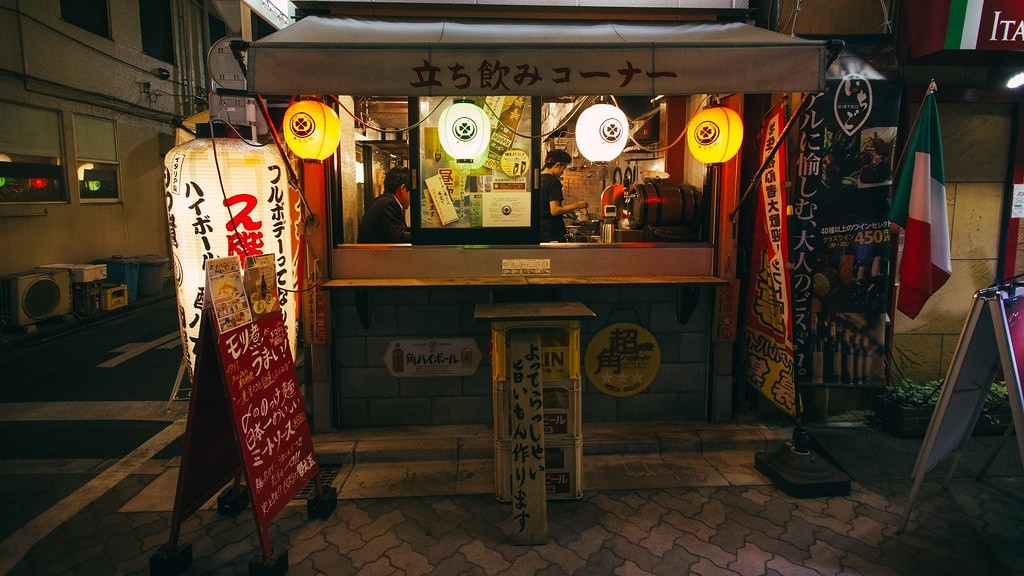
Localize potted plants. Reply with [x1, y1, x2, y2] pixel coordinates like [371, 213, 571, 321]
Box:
[879, 374, 1014, 439]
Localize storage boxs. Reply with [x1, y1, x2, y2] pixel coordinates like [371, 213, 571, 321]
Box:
[100, 283, 129, 310]
[74, 282, 100, 316]
[37, 263, 108, 281]
[492, 319, 583, 500]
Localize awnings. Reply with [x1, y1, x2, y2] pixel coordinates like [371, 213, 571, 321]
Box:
[230, 13, 828, 220]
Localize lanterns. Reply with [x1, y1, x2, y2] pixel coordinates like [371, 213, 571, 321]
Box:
[437, 99, 491, 163]
[575, 100, 629, 167]
[162, 121, 299, 383]
[687, 102, 745, 167]
[283, 95, 342, 164]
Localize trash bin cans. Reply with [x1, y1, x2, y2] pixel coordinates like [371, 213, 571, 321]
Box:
[93, 255, 140, 306]
[137, 254, 170, 301]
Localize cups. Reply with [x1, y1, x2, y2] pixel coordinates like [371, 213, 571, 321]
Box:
[461, 347, 473, 368]
[256, 279, 262, 301]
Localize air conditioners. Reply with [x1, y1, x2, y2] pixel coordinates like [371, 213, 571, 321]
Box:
[2, 269, 74, 325]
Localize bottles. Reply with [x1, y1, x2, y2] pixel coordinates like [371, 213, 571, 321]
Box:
[601, 217, 616, 242]
[261, 274, 266, 298]
[393, 343, 404, 372]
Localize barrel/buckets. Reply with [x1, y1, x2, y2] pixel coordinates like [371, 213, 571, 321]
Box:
[628, 182, 702, 230]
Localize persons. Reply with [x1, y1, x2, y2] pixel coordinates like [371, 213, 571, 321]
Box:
[541, 150, 589, 243]
[357, 167, 411, 244]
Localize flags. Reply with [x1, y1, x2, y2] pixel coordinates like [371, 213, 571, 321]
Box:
[891, 93, 953, 320]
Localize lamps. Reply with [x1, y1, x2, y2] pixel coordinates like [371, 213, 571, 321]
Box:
[826, 39, 888, 83]
